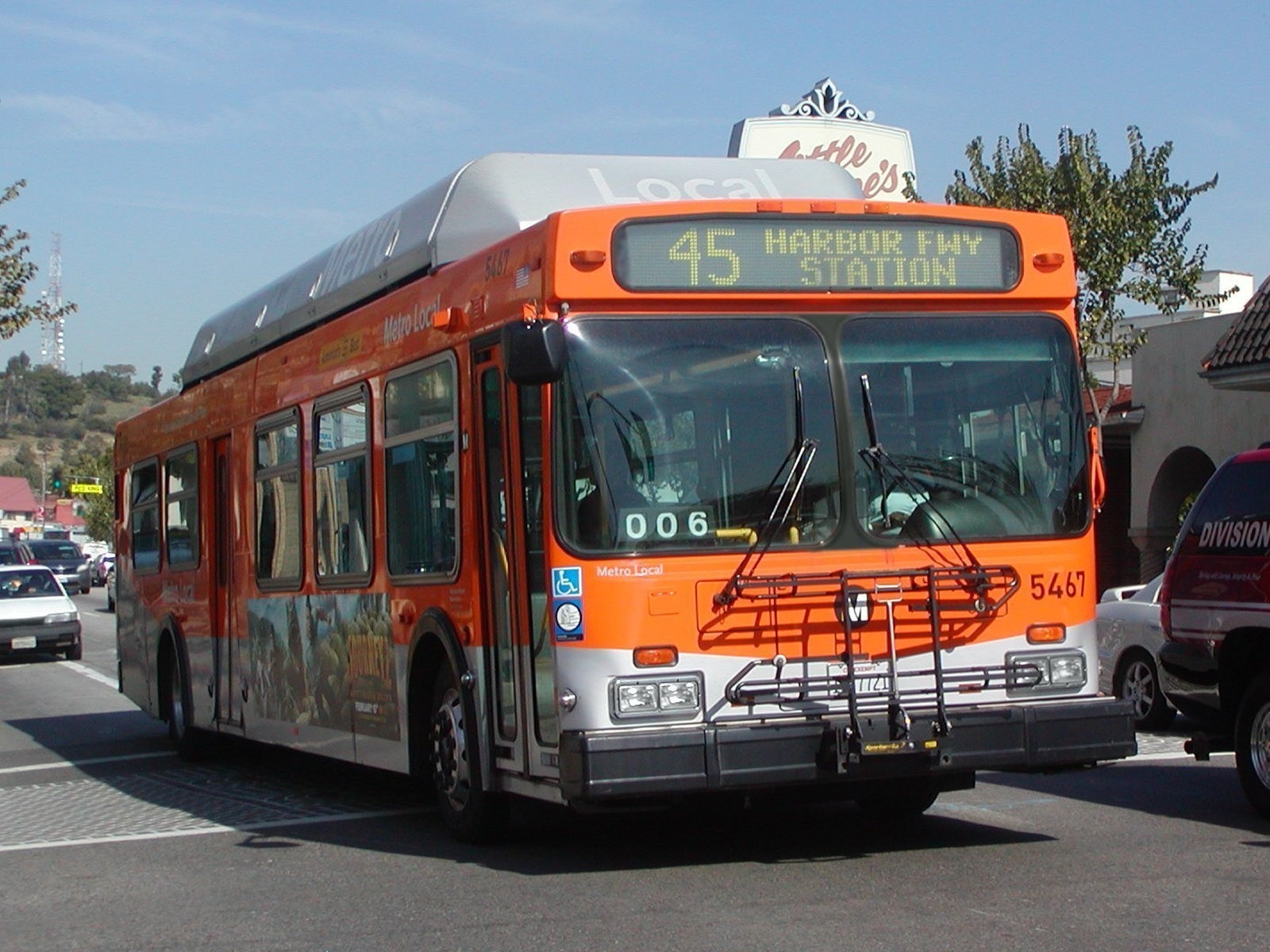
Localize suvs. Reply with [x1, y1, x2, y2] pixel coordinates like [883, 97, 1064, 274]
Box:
[1155, 437, 1270, 816]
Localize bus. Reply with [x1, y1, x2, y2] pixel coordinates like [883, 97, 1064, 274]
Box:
[117, 114, 1143, 842]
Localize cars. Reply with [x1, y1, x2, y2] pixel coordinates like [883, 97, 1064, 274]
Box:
[0, 562, 82, 664]
[0, 541, 38, 566]
[29, 539, 91, 596]
[1093, 573, 1166, 732]
[88, 552, 118, 612]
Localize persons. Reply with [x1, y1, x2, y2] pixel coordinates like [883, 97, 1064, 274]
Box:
[867, 428, 971, 535]
[9, 576, 45, 596]
[579, 456, 651, 544]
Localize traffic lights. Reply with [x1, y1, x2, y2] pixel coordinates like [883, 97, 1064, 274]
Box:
[53, 469, 61, 489]
